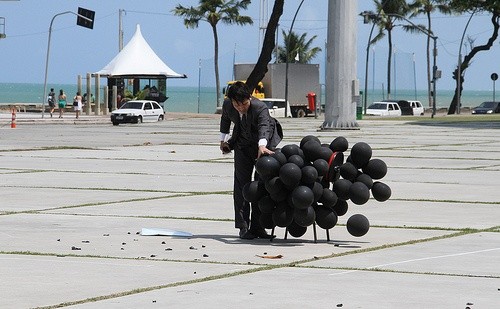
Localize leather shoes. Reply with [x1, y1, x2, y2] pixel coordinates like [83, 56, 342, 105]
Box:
[251, 227, 277, 239]
[239, 228, 257, 239]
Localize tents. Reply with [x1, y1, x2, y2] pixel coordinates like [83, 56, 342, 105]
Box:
[90, 24, 188, 78]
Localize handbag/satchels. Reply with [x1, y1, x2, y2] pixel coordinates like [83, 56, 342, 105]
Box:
[73, 102, 78, 106]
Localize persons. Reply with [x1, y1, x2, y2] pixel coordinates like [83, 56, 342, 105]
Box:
[219, 81, 284, 239]
[49, 88, 122, 119]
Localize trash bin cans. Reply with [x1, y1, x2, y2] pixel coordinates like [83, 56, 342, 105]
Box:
[308, 92, 315, 110]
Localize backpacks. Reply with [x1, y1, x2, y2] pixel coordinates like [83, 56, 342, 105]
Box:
[48, 93, 54, 102]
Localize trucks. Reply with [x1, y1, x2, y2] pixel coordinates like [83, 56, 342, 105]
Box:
[222, 62, 321, 119]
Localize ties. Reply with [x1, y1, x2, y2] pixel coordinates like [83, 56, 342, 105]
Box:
[241, 114, 247, 125]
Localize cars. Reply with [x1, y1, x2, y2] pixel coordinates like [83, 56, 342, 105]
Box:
[382, 99, 414, 115]
[110, 99, 165, 126]
[408, 101, 425, 116]
[471, 101, 500, 114]
[362, 101, 401, 116]
[258, 98, 292, 118]
